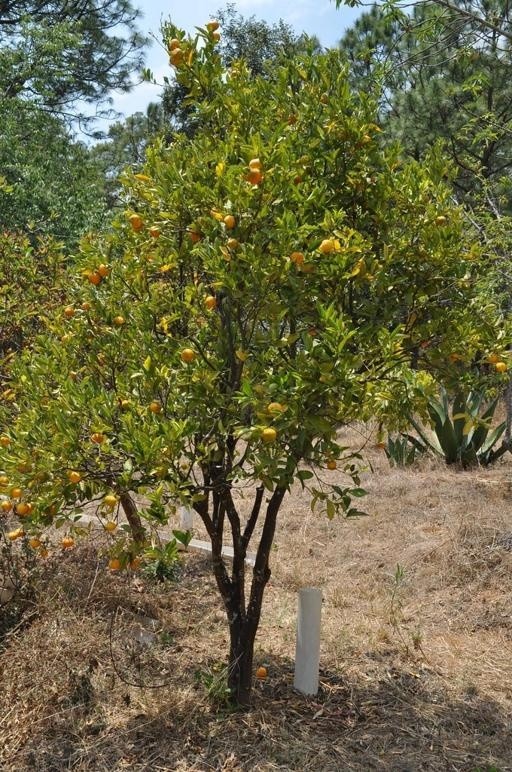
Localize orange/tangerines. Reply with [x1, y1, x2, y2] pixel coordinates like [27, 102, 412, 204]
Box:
[0, 20, 508, 571]
[256, 667, 267, 677]
